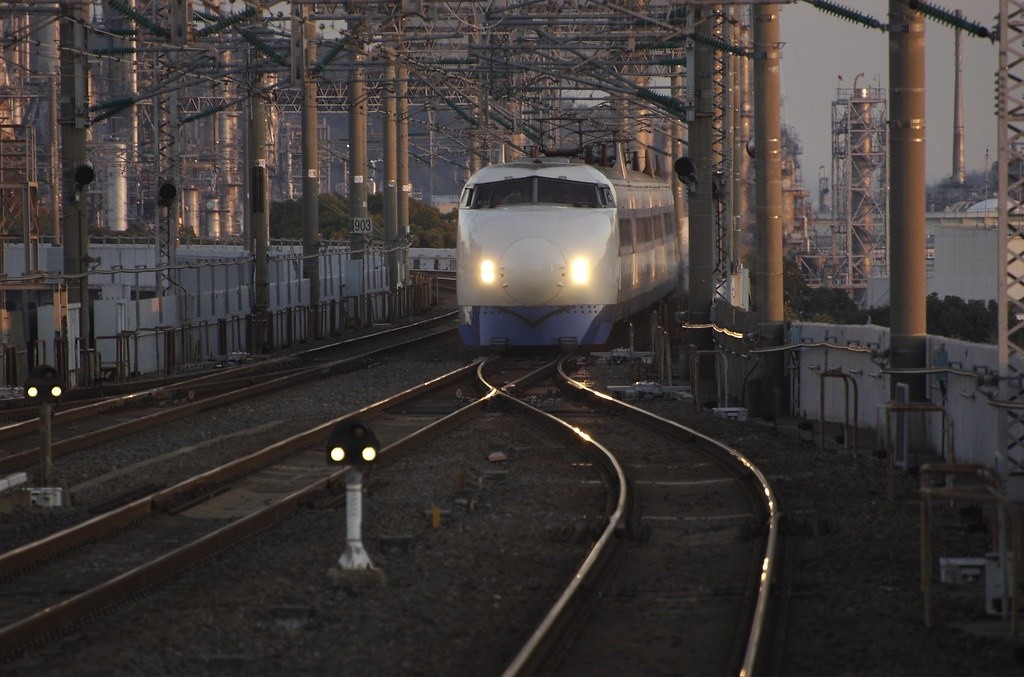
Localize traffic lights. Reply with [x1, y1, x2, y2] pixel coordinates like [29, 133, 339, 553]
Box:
[327, 423, 380, 464]
[25, 363, 67, 405]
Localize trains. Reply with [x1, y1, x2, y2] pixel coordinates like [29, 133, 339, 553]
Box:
[458, 116, 723, 352]
